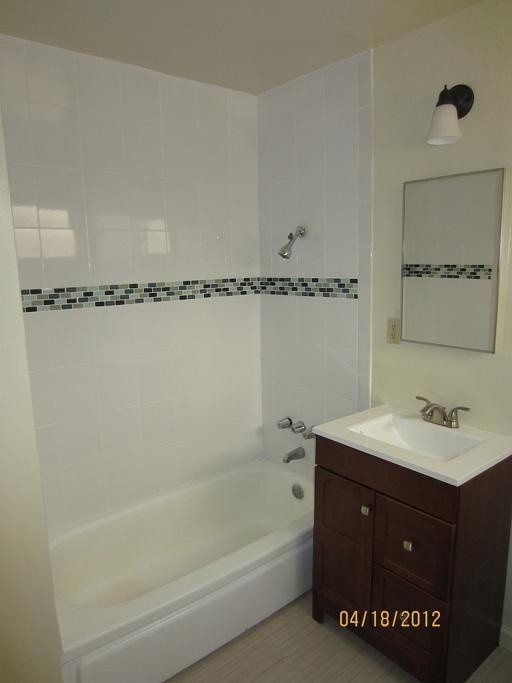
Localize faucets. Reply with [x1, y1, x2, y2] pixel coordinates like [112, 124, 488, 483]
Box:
[422, 404, 447, 426]
[281, 446, 305, 464]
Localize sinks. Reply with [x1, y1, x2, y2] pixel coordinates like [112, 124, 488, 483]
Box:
[347, 413, 486, 461]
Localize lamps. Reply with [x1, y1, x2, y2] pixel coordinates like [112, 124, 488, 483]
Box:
[426, 83, 474, 147]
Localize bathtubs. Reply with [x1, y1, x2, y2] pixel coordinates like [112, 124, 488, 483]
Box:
[46, 460, 314, 682]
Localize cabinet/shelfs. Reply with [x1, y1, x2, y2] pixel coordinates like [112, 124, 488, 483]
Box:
[311, 436, 511, 683]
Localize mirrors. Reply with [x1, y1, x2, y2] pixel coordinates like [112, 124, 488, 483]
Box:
[399, 167, 505, 353]
